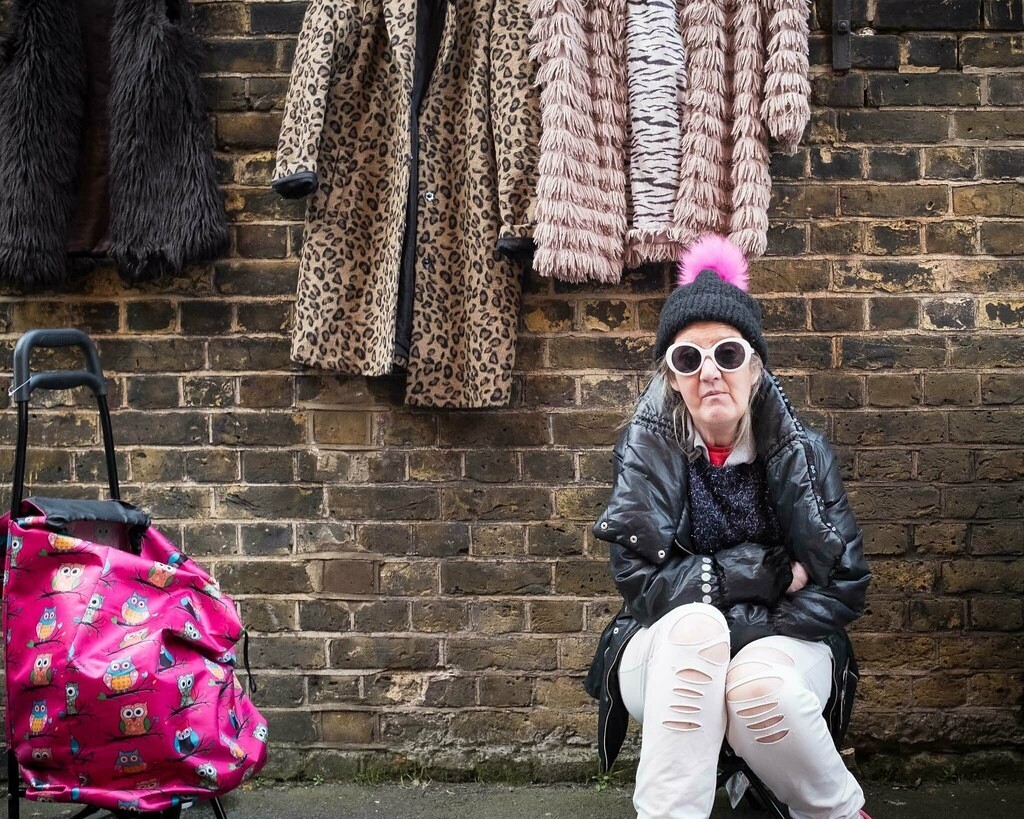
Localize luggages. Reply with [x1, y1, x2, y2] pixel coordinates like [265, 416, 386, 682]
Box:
[3, 328, 273, 818]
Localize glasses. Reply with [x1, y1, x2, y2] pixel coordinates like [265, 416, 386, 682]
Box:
[665, 340, 755, 375]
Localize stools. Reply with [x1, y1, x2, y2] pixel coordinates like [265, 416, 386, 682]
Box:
[716, 735, 793, 819]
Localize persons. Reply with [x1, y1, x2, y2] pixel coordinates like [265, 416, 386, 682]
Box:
[580, 232, 874, 819]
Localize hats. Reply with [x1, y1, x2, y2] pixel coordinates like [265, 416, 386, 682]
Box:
[651, 236, 769, 358]
